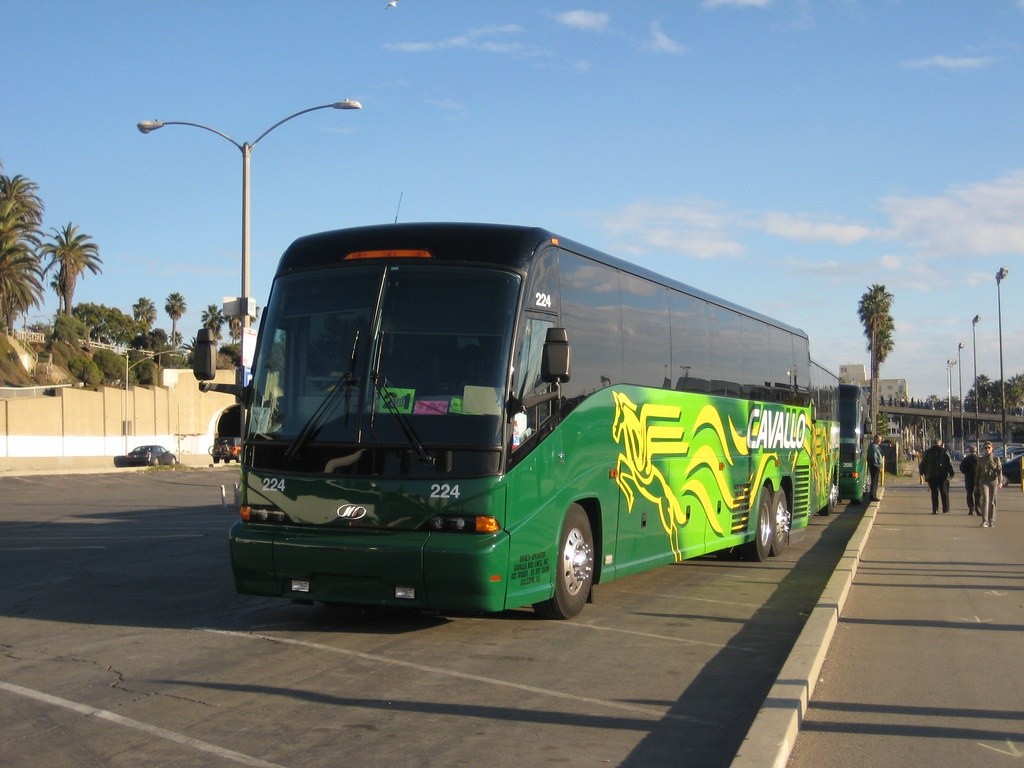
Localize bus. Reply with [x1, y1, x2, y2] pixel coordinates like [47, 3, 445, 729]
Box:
[810, 357, 841, 523]
[839, 385, 871, 504]
[193, 208, 816, 623]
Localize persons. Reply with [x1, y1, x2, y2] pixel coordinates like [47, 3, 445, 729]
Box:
[974, 441, 1002, 527]
[866, 435, 882, 502]
[959, 444, 981, 516]
[912, 448, 916, 461]
[919, 437, 955, 515]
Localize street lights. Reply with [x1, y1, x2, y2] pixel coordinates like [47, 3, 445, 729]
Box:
[123, 348, 193, 456]
[943, 358, 956, 449]
[957, 343, 966, 449]
[995, 267, 1011, 454]
[133, 98, 364, 377]
[210, 437, 242, 465]
[972, 315, 984, 442]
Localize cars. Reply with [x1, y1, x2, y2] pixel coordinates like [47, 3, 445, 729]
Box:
[113, 445, 177, 467]
[956, 440, 1024, 488]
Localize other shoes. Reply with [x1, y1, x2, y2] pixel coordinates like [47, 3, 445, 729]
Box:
[967, 509, 981, 516]
[981, 522, 994, 528]
[869, 498, 880, 502]
[932, 510, 938, 514]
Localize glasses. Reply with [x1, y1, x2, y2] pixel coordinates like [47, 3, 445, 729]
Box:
[984, 447, 992, 450]
